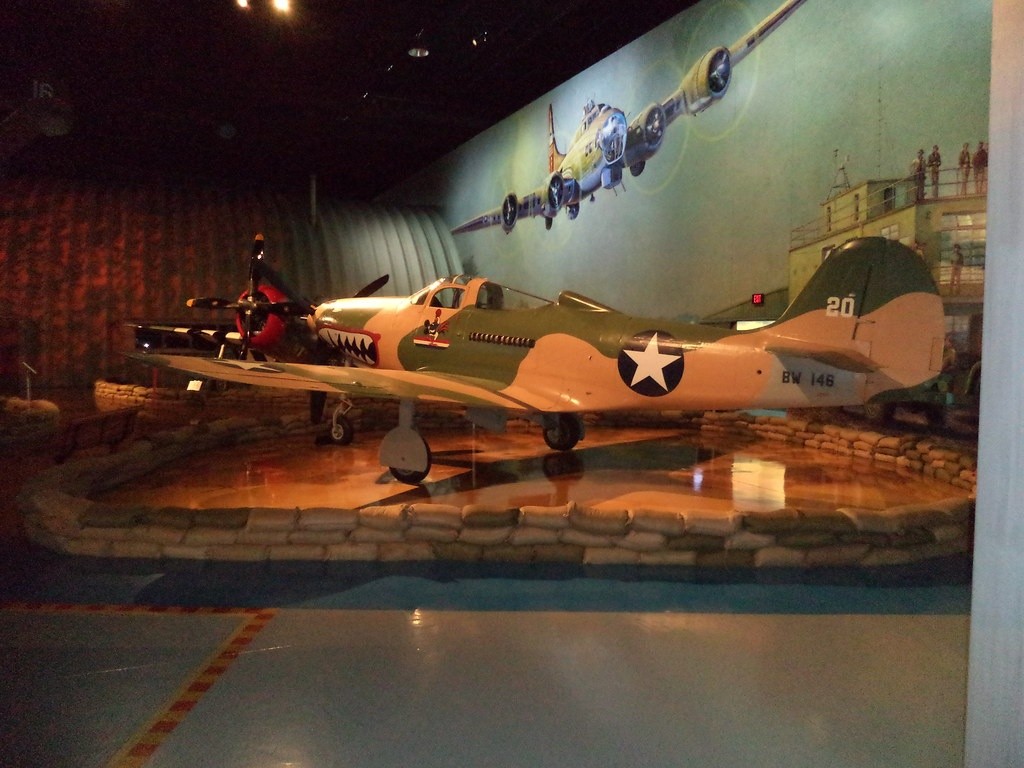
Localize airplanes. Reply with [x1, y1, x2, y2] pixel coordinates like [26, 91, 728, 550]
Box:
[450, 0, 807, 233]
[122, 235, 945, 486]
[128, 232, 390, 427]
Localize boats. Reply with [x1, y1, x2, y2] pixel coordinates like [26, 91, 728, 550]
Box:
[699, 53, 991, 413]
